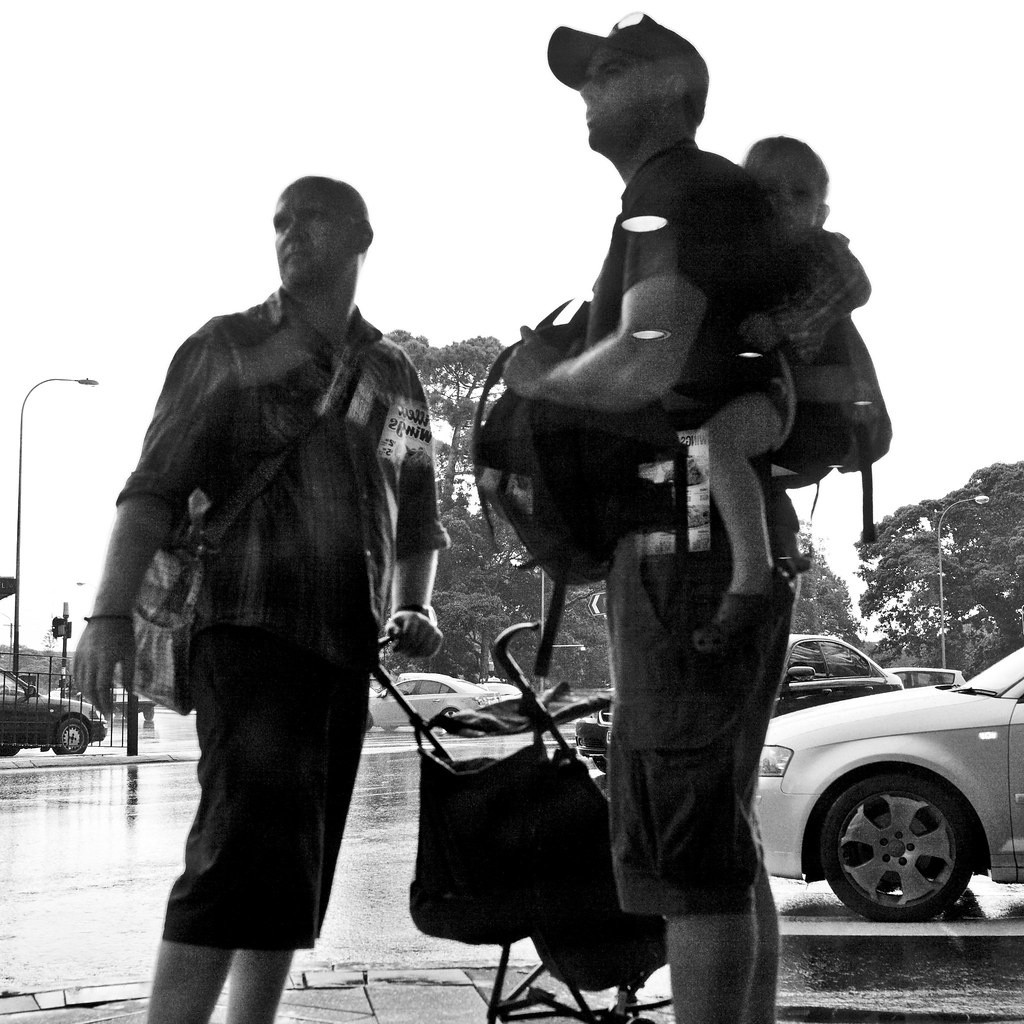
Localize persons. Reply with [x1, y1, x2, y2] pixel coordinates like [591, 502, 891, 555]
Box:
[504, 12, 814, 1024]
[690, 136, 873, 652]
[74, 176, 451, 1024]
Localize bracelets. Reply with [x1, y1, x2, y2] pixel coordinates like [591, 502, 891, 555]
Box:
[84, 614, 133, 622]
[394, 604, 437, 627]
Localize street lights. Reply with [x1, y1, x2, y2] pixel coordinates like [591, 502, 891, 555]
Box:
[938, 495, 989, 670]
[13, 378, 99, 675]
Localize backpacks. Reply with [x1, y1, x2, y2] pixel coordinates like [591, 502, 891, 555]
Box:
[479, 297, 648, 586]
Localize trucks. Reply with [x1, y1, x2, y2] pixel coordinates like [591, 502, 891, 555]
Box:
[110, 681, 157, 721]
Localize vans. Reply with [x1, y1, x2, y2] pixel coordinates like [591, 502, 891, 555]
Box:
[879, 667, 966, 690]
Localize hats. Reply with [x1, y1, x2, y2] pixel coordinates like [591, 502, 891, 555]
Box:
[547, 11, 709, 123]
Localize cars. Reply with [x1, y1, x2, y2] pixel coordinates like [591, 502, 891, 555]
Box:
[575, 635, 904, 773]
[366, 677, 501, 731]
[753, 644, 1024, 922]
[475, 683, 523, 699]
[0, 667, 108, 758]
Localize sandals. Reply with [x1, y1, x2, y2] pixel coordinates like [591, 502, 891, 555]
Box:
[691, 562, 797, 661]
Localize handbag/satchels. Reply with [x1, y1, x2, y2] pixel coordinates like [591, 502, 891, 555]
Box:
[111, 543, 208, 716]
